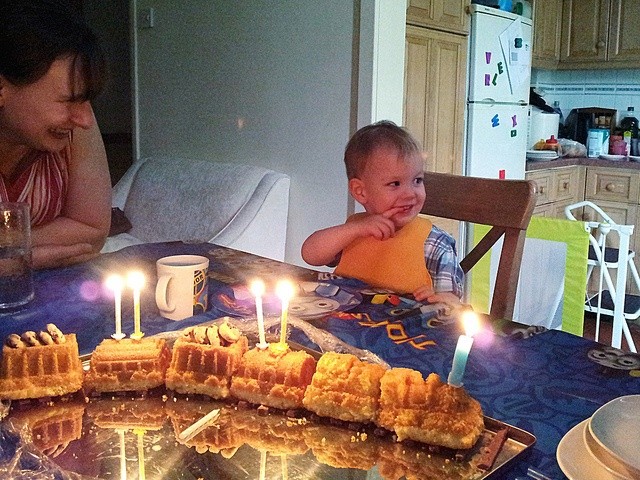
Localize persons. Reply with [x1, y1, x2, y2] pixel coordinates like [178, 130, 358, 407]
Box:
[297, 119, 464, 311]
[1, 1, 114, 274]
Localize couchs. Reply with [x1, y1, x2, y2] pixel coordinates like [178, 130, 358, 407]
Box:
[101, 154, 292, 264]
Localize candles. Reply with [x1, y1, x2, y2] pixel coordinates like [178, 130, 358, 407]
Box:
[445, 333, 475, 389]
[270, 296, 294, 354]
[128, 289, 145, 340]
[107, 289, 127, 342]
[250, 294, 270, 349]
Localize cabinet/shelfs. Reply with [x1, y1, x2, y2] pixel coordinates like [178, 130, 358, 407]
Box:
[404, 1, 469, 258]
[524, 166, 581, 220]
[581, 166, 640, 296]
[554, 0, 640, 72]
[531, 0, 562, 71]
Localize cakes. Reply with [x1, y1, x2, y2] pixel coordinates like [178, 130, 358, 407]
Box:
[0, 321, 84, 400]
[302, 349, 383, 423]
[376, 371, 483, 450]
[231, 343, 316, 408]
[90, 337, 170, 394]
[165, 322, 248, 400]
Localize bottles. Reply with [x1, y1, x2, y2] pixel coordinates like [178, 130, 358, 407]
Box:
[553, 100, 563, 132]
[545, 135, 559, 157]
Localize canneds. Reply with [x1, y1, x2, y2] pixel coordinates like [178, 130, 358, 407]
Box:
[586, 129, 610, 158]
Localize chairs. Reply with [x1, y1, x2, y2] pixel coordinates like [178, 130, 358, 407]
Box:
[565, 200, 640, 353]
[419, 169, 537, 321]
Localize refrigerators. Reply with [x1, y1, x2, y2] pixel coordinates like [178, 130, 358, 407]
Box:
[460, 3, 535, 319]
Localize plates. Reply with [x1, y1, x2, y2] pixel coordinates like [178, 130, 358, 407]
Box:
[600, 153, 626, 162]
[526, 150, 561, 161]
[556, 416, 640, 480]
[627, 154, 640, 162]
[582, 421, 640, 480]
[588, 394, 640, 472]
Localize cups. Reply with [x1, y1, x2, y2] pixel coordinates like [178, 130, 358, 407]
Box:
[0, 203, 36, 309]
[154, 254, 210, 320]
